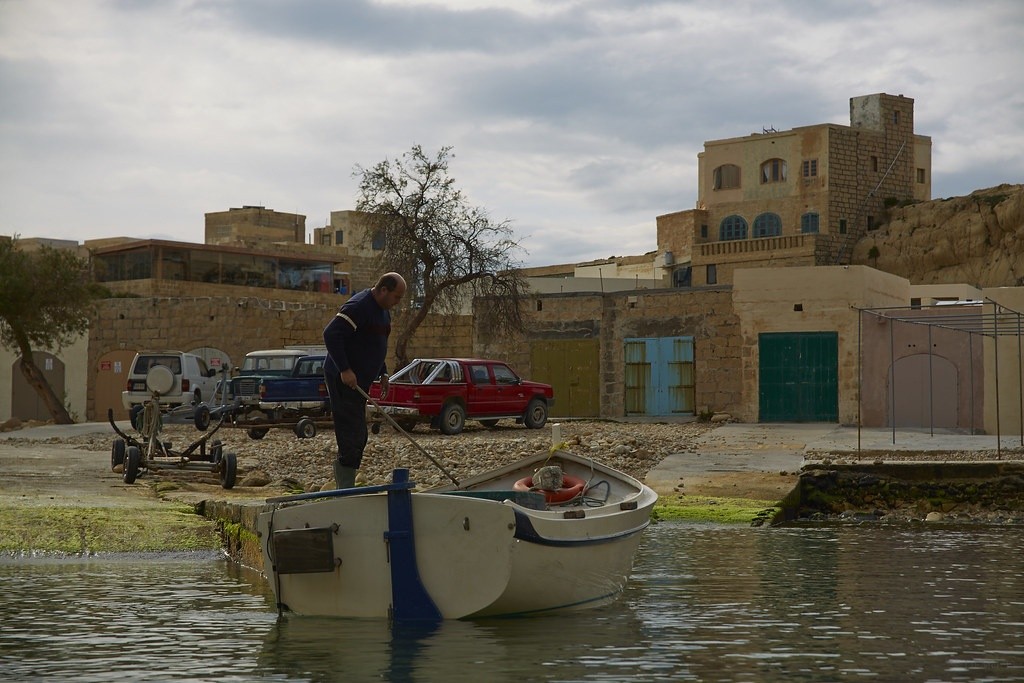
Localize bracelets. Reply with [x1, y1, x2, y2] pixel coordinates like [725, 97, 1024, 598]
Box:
[381, 374, 389, 380]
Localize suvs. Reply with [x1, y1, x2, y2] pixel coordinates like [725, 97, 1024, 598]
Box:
[120, 351, 217, 431]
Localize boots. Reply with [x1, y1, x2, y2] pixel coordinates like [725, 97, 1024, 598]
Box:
[332, 460, 355, 490]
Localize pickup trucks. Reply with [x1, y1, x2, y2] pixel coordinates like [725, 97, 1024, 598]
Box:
[230, 355, 331, 423]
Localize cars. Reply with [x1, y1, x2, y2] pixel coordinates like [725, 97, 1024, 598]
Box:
[369, 358, 555, 435]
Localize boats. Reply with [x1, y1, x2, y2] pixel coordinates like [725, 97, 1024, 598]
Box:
[260, 422, 660, 625]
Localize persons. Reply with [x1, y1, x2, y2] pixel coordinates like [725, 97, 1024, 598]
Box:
[322, 272, 407, 489]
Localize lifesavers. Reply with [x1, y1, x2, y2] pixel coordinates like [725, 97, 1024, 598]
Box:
[512, 471, 586, 505]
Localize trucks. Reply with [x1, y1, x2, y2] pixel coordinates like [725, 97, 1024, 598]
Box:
[235, 345, 324, 377]
